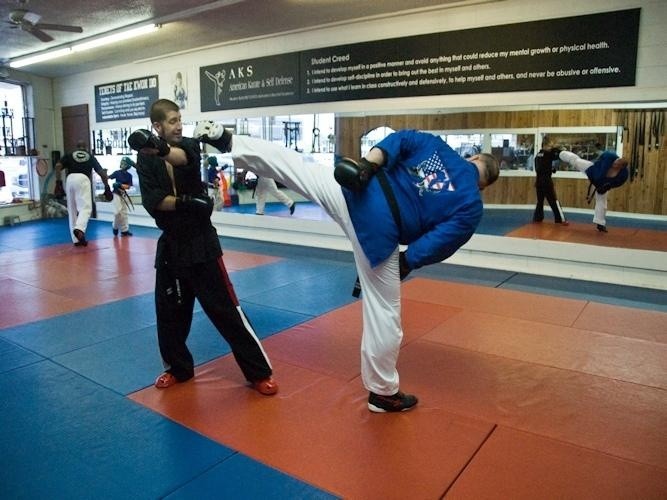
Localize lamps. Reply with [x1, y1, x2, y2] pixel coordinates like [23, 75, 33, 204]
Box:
[7, 19, 164, 69]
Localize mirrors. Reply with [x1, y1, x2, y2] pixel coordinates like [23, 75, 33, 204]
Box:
[535, 124, 623, 180]
[484, 127, 537, 178]
[416, 128, 483, 159]
[91, 84, 665, 273]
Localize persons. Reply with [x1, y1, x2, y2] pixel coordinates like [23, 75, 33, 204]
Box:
[128, 99, 278, 396]
[233, 168, 295, 215]
[208, 155, 228, 211]
[194, 120, 500, 414]
[109, 156, 133, 236]
[552, 148, 629, 233]
[533, 140, 568, 226]
[55, 140, 113, 246]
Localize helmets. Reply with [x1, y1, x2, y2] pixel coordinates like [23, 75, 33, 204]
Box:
[120, 157, 131, 169]
[207, 157, 218, 166]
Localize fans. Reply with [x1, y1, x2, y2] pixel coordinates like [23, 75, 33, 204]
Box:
[0, 0, 83, 44]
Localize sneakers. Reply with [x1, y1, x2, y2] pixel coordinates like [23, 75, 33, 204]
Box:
[113, 229, 118, 236]
[255, 376, 278, 395]
[193, 120, 232, 153]
[290, 202, 295, 214]
[155, 373, 177, 389]
[74, 229, 88, 247]
[121, 231, 133, 237]
[368, 391, 418, 413]
[597, 224, 608, 233]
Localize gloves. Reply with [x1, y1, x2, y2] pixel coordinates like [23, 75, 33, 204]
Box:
[55, 180, 66, 200]
[222, 164, 228, 170]
[334, 157, 378, 192]
[175, 194, 214, 216]
[103, 185, 113, 201]
[128, 129, 170, 157]
